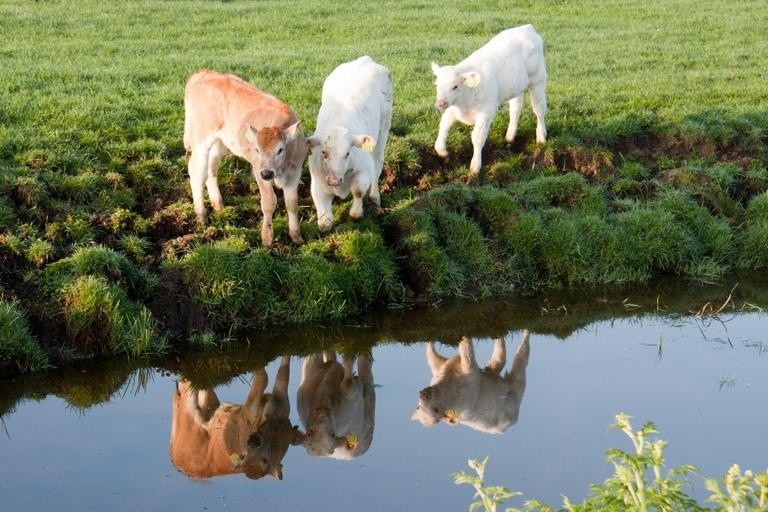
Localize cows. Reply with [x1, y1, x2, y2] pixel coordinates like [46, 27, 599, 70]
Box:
[181, 68, 308, 248]
[304, 53, 395, 233]
[165, 355, 303, 483]
[409, 327, 532, 436]
[295, 350, 378, 461]
[427, 22, 551, 177]
